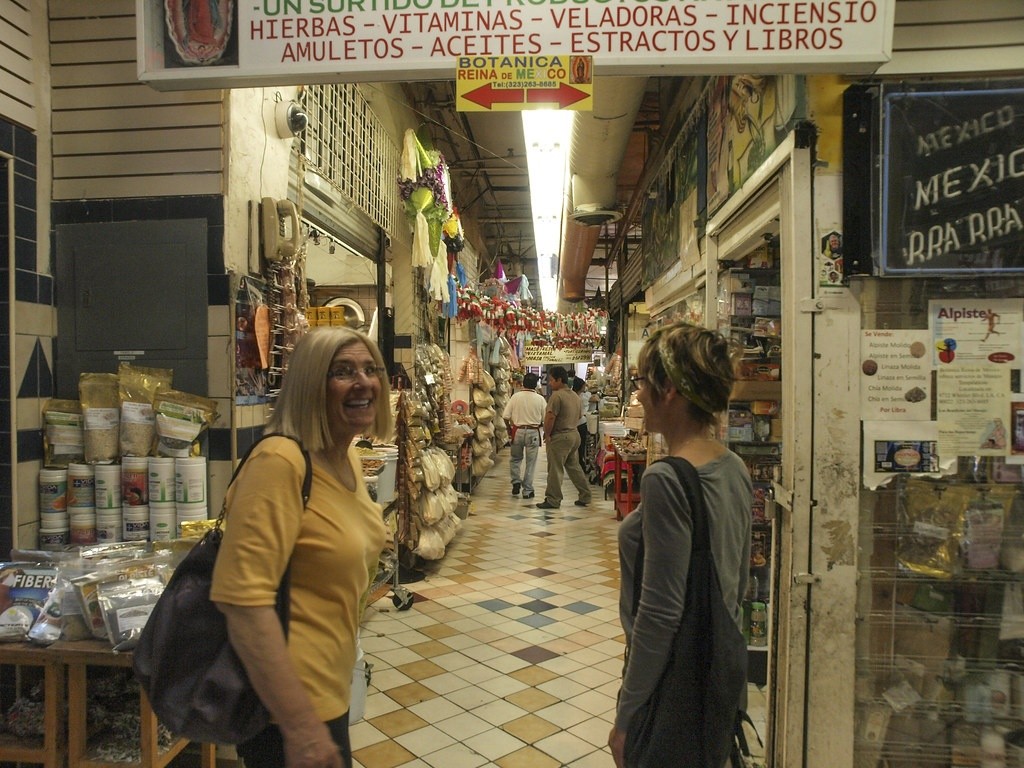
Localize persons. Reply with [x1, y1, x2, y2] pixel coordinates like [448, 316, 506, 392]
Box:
[502, 365, 601, 509]
[607, 322, 754, 768]
[209, 326, 392, 768]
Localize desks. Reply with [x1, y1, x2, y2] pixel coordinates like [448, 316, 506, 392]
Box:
[0, 635, 218, 768]
[595, 441, 646, 522]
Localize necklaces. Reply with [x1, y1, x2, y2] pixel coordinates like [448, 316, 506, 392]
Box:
[325, 452, 357, 492]
[672, 433, 714, 456]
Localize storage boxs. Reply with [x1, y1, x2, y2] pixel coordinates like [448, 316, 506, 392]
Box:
[598, 395, 621, 417]
[587, 351, 606, 376]
[714, 266, 781, 455]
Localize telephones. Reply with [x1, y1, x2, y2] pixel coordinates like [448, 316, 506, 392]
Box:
[261, 196, 301, 262]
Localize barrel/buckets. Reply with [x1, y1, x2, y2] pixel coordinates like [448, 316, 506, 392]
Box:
[373, 442, 400, 502]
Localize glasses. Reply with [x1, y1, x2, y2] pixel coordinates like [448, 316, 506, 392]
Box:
[631, 375, 645, 390]
[327, 365, 384, 383]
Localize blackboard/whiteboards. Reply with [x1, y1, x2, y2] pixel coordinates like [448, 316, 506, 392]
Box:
[871, 69, 1024, 280]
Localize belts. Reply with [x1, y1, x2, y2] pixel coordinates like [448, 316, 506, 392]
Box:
[518, 426, 538, 429]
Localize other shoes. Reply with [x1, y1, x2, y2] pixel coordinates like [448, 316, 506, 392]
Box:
[512, 483, 520, 494]
[575, 500, 589, 505]
[536, 498, 556, 508]
[523, 491, 534, 498]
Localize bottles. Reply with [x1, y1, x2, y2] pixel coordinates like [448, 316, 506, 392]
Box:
[38, 457, 208, 548]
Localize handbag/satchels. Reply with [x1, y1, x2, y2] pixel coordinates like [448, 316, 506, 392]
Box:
[132, 432, 313, 746]
[615, 456, 747, 767]
